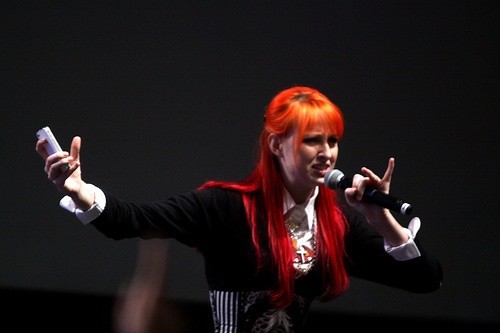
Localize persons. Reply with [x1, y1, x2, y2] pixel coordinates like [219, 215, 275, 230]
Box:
[34, 85, 444, 332]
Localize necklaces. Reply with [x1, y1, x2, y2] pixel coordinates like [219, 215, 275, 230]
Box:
[284, 214, 317, 275]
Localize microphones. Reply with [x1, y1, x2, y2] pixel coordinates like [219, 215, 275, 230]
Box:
[324, 168, 413, 218]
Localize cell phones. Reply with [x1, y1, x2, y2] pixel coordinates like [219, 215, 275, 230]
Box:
[36, 127, 69, 176]
